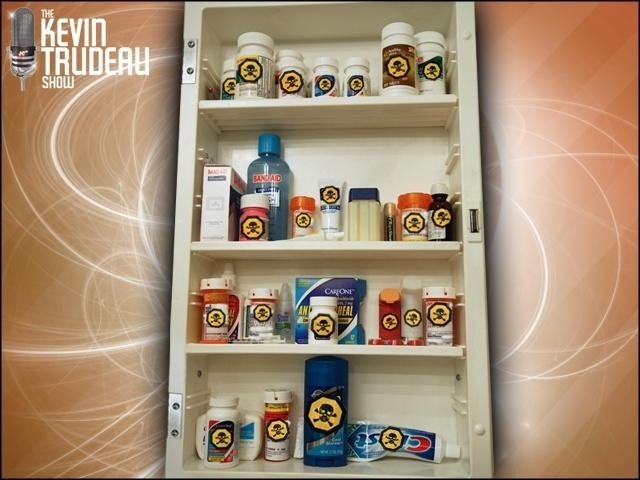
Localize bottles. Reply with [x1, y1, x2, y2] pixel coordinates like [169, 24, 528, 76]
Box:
[343, 56, 372, 97]
[233, 31, 281, 102]
[309, 55, 340, 100]
[194, 415, 208, 460]
[415, 31, 450, 95]
[300, 356, 351, 470]
[274, 48, 309, 101]
[198, 131, 457, 344]
[205, 393, 241, 471]
[263, 389, 292, 462]
[379, 20, 417, 94]
[220, 59, 236, 101]
[233, 412, 263, 462]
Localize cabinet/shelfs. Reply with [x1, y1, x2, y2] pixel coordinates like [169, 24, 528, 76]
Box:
[158, 2, 497, 480]
